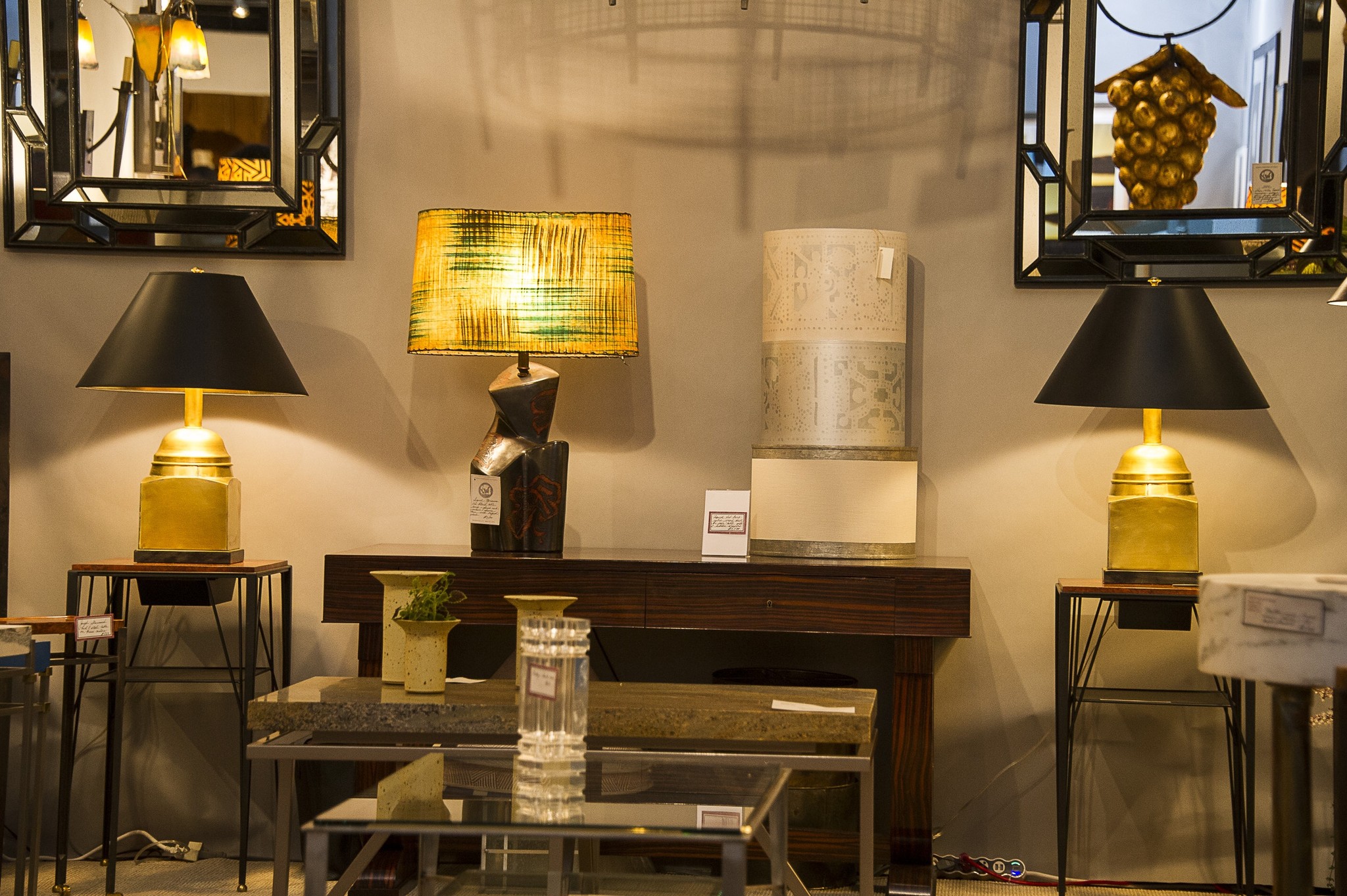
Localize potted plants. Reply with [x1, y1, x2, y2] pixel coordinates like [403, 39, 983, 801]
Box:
[392, 569, 467, 692]
[406, 209, 639, 553]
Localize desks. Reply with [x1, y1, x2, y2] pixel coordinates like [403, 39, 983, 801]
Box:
[300, 749, 792, 896]
[323, 542, 972, 896]
[1195, 572, 1347, 896]
[244, 675, 879, 896]
[1053, 579, 1255, 896]
[0, 558, 293, 896]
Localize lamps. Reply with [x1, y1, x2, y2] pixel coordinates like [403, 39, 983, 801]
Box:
[77, 1, 212, 181]
[71, 267, 310, 566]
[217, 156, 272, 182]
[1033, 274, 1270, 584]
[231, 0, 251, 18]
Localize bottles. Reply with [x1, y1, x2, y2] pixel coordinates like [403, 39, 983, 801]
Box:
[516, 617, 593, 763]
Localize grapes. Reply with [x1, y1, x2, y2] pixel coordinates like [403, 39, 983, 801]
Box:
[1107, 65, 1216, 209]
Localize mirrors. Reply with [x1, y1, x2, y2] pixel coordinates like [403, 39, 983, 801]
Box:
[1012, 0, 1347, 290]
[0, 0, 347, 256]
